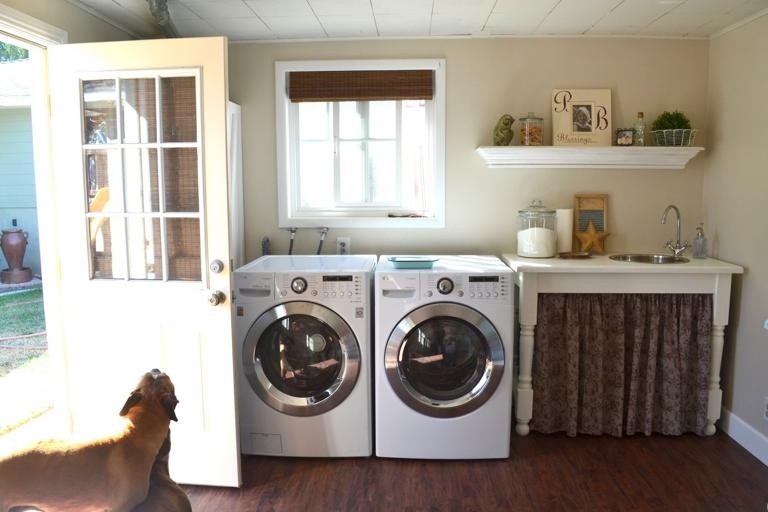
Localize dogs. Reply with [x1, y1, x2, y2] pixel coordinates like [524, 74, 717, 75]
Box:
[0, 366, 193, 512]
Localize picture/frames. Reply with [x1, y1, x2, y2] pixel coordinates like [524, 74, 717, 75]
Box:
[550, 88, 612, 147]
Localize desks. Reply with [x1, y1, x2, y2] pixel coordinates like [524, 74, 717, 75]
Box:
[498, 249, 746, 436]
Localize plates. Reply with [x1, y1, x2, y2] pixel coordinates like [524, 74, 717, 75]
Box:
[558, 251, 591, 259]
[387, 255, 439, 270]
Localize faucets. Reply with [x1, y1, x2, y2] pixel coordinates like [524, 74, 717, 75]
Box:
[660, 204, 682, 247]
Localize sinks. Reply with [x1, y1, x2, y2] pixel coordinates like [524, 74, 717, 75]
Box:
[609, 253, 690, 262]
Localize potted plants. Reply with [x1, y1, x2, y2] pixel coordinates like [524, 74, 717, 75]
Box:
[646, 109, 698, 146]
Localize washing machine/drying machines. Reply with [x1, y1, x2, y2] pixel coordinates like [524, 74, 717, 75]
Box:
[374, 254, 515, 460]
[231, 254, 376, 458]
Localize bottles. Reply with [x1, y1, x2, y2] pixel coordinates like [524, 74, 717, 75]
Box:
[519, 112, 544, 146]
[515, 200, 559, 259]
[635, 111, 647, 146]
